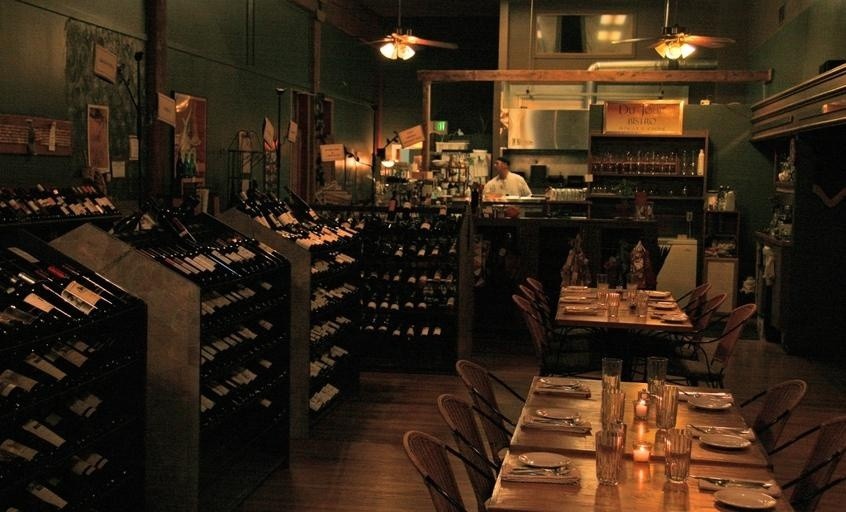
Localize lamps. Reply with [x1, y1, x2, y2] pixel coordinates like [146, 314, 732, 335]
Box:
[655, 38, 695, 59]
[379, 40, 416, 61]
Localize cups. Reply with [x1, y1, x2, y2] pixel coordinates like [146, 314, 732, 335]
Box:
[601, 357, 622, 392]
[665, 428, 692, 482]
[656, 386, 679, 429]
[602, 391, 626, 424]
[595, 430, 620, 486]
[596, 274, 649, 318]
[647, 356, 668, 394]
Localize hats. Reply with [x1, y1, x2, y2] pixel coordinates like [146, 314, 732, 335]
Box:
[497, 157, 510, 165]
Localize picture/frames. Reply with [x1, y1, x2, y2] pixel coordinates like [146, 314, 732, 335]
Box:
[172, 92, 208, 214]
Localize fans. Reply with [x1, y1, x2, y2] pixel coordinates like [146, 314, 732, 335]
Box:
[611, 0, 737, 50]
[361, 1, 458, 52]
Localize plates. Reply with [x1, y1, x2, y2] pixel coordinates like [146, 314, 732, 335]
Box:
[537, 409, 582, 420]
[700, 435, 751, 449]
[714, 490, 776, 510]
[519, 451, 572, 468]
[560, 285, 688, 322]
[689, 397, 732, 409]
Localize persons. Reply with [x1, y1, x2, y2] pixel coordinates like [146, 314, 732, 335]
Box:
[482, 158, 532, 197]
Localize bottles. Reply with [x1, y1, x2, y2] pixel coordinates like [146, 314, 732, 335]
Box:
[784, 205, 794, 240]
[718, 185, 735, 211]
[2, 182, 113, 231]
[234, 182, 352, 434]
[109, 207, 289, 511]
[0, 237, 151, 510]
[353, 193, 472, 360]
[590, 148, 704, 195]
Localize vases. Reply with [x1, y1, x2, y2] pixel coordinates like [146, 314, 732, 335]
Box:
[777, 162, 795, 188]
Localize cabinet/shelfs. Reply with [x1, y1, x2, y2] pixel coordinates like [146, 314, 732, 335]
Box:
[587, 130, 741, 316]
[311, 200, 474, 378]
[753, 230, 795, 327]
[475, 215, 656, 342]
[773, 146, 796, 194]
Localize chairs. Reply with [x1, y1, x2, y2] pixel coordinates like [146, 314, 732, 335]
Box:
[511, 275, 758, 391]
